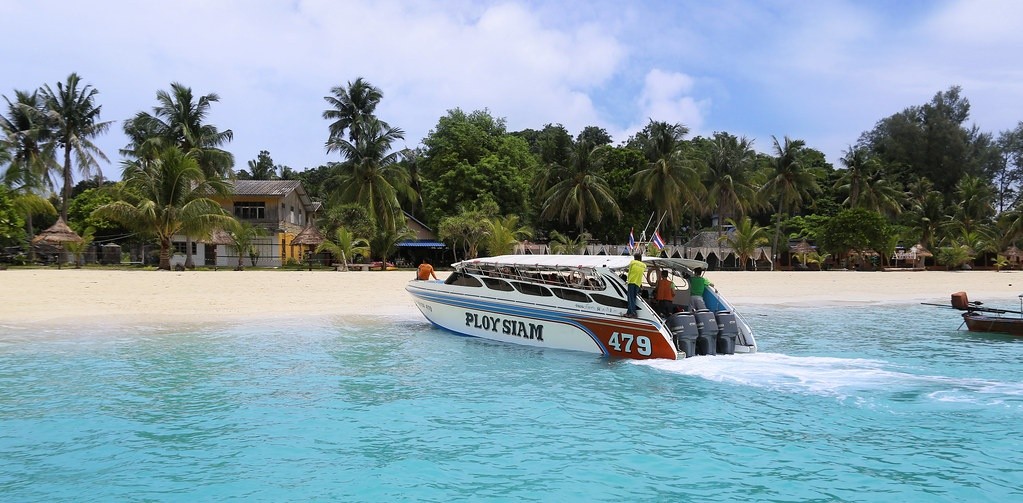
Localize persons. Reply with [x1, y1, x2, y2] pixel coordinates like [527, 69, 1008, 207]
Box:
[622, 253, 646, 317]
[414, 257, 439, 280]
[686, 267, 718, 312]
[655, 270, 675, 318]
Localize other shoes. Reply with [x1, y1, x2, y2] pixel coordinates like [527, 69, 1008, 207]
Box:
[624, 313, 637, 319]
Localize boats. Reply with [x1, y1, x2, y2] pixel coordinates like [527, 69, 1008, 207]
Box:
[403, 252, 759, 363]
[950, 291, 1023, 336]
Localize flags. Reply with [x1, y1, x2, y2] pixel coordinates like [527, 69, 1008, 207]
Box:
[652, 227, 665, 249]
[629, 227, 634, 249]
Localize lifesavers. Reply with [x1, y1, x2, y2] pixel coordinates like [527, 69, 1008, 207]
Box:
[646, 266, 663, 286]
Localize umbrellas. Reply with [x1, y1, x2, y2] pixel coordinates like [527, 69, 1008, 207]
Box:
[906, 243, 933, 268]
[1000, 246, 1023, 269]
[195, 226, 237, 266]
[289, 222, 329, 271]
[31, 215, 83, 269]
[789, 241, 816, 265]
